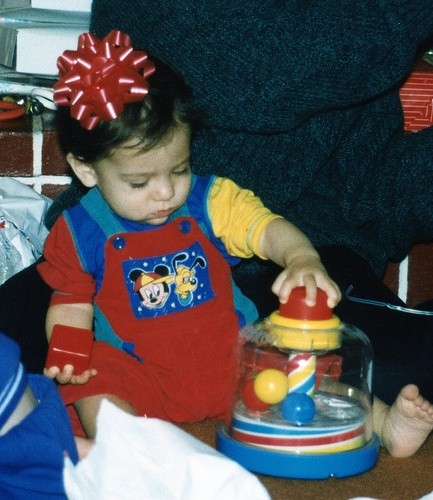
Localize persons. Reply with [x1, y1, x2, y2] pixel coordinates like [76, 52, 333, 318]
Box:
[1, 0, 432, 407]
[1, 336, 78, 500]
[40, 47, 433, 462]
